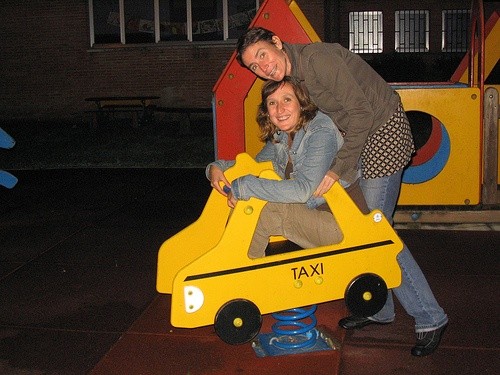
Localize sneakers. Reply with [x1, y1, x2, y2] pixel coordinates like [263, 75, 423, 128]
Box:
[412, 322, 448, 357]
[338, 314, 392, 330]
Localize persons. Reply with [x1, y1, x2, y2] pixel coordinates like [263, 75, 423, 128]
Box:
[235, 26, 448, 358]
[205, 76, 371, 261]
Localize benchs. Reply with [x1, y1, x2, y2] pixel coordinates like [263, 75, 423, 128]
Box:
[85, 105, 213, 129]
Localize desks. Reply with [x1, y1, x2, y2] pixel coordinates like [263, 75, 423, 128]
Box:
[84, 96, 160, 128]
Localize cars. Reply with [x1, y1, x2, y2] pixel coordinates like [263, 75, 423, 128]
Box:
[155, 153, 404, 345]
